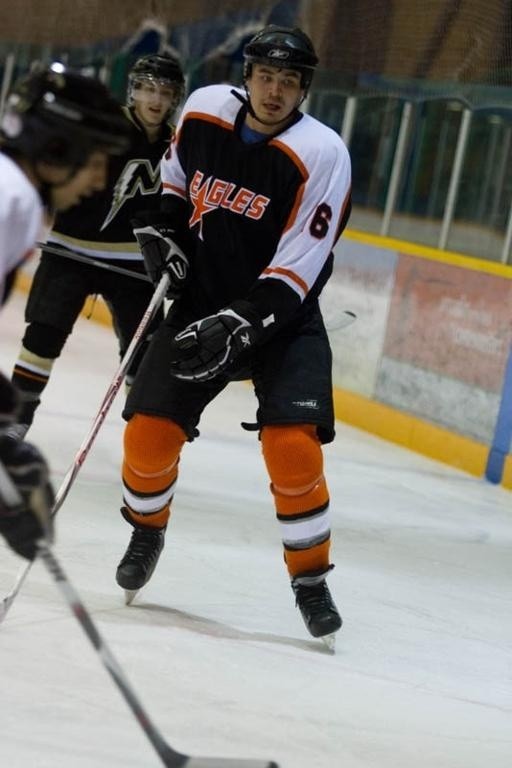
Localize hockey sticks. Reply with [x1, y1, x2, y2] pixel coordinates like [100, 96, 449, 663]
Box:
[0, 270, 172, 617]
[0, 462, 283, 767]
[35, 242, 358, 333]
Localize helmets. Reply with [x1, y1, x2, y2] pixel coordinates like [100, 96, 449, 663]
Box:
[242, 24, 318, 91]
[127, 51, 189, 111]
[0, 61, 144, 189]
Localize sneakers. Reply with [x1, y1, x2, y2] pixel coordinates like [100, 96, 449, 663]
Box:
[291, 563, 342, 637]
[117, 507, 169, 589]
[0, 398, 43, 453]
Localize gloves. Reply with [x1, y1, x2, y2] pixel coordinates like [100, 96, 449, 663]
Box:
[169, 292, 282, 384]
[0, 441, 56, 560]
[128, 209, 203, 300]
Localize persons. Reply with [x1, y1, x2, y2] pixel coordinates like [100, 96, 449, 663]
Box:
[114, 29, 352, 636]
[0, 69, 131, 562]
[1, 55, 185, 446]
